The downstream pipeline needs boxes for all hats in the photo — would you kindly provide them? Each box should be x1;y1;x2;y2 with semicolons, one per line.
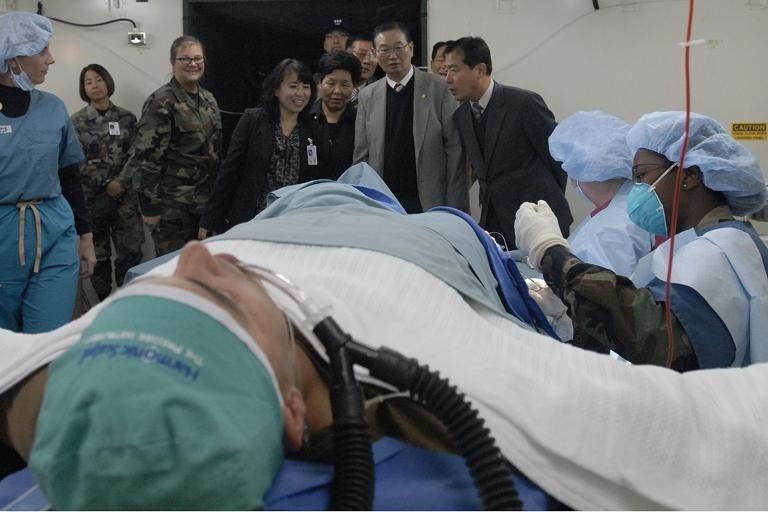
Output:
323;17;353;36
27;283;286;511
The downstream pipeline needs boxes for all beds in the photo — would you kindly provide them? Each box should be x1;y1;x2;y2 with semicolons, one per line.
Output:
0;251;555;511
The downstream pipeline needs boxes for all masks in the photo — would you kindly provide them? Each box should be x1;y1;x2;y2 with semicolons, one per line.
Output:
7;56;34;92
572;180;597;210
626;162;679;239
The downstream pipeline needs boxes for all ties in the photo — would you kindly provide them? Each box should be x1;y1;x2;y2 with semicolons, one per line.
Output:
472;102;483;120
394;83;404;93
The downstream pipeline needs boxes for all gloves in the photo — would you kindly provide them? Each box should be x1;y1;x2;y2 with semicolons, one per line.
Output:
524;277;568;321
514;199;570;274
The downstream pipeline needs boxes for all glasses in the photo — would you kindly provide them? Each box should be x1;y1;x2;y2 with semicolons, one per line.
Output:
352;48;376;59
374;42;410;54
176;55;207;65
325;32;350;40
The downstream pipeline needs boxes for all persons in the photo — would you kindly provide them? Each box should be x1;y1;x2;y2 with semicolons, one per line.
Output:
69;63;146;305
319;16;354;58
430;38;478;222
353;18;462;216
344;30;384;107
548;111;668;282
133;35;224;260
305;50;363;187
0;13;98;335
222;57;327;236
8;238;457;510
442;35;574;252
512;110;768;374
